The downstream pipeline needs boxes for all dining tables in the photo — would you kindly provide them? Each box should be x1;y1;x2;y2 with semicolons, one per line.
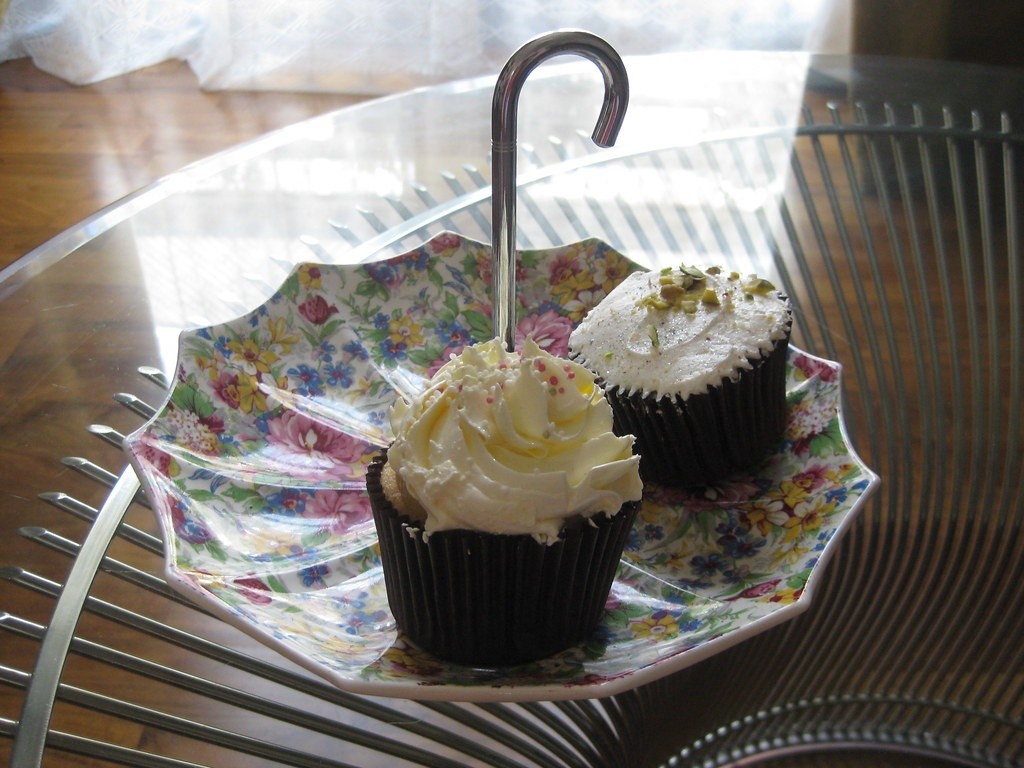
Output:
0;38;1020;766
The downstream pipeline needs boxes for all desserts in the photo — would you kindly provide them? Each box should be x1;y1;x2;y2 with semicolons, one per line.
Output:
566;264;795;490
380;333;643;667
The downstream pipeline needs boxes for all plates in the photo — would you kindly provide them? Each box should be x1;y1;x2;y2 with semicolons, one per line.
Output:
125;221;885;705
